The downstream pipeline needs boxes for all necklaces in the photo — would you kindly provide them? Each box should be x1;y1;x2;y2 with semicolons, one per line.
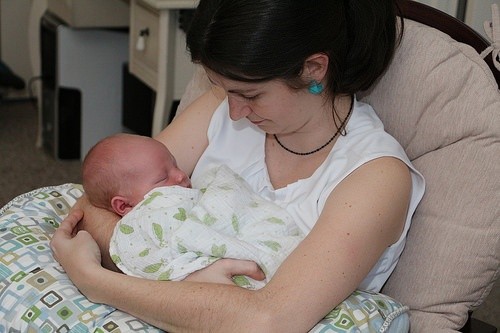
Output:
274;95;355;155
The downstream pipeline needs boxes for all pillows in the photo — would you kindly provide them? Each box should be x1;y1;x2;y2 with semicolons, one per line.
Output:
0;184;411;332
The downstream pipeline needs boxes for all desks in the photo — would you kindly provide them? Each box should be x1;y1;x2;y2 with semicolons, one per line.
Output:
27;0;199;161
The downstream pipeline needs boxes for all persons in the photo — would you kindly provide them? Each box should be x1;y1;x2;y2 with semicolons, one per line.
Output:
49;0;426;333
81;132;308;289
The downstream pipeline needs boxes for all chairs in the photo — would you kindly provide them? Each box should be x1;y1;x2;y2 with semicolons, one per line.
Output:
172;0;500;333
